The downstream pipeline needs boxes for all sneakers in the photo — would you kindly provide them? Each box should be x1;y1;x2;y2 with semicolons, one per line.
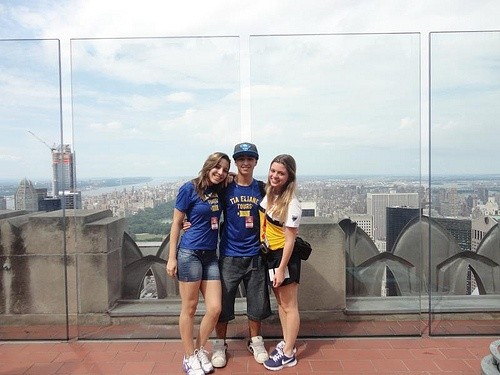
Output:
211;338;228;367
263;349;298;370
182;349;205;375
249;335;268;364
269;340;296;359
195;346;214;373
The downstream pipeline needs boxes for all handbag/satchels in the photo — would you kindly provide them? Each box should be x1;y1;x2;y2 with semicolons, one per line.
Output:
295;237;312;261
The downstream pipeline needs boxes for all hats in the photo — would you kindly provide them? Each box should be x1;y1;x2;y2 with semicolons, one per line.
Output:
233;142;259;159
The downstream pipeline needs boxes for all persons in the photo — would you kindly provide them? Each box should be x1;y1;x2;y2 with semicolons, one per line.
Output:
182;143;298;368
229;154;301;370
166;152;233;375
